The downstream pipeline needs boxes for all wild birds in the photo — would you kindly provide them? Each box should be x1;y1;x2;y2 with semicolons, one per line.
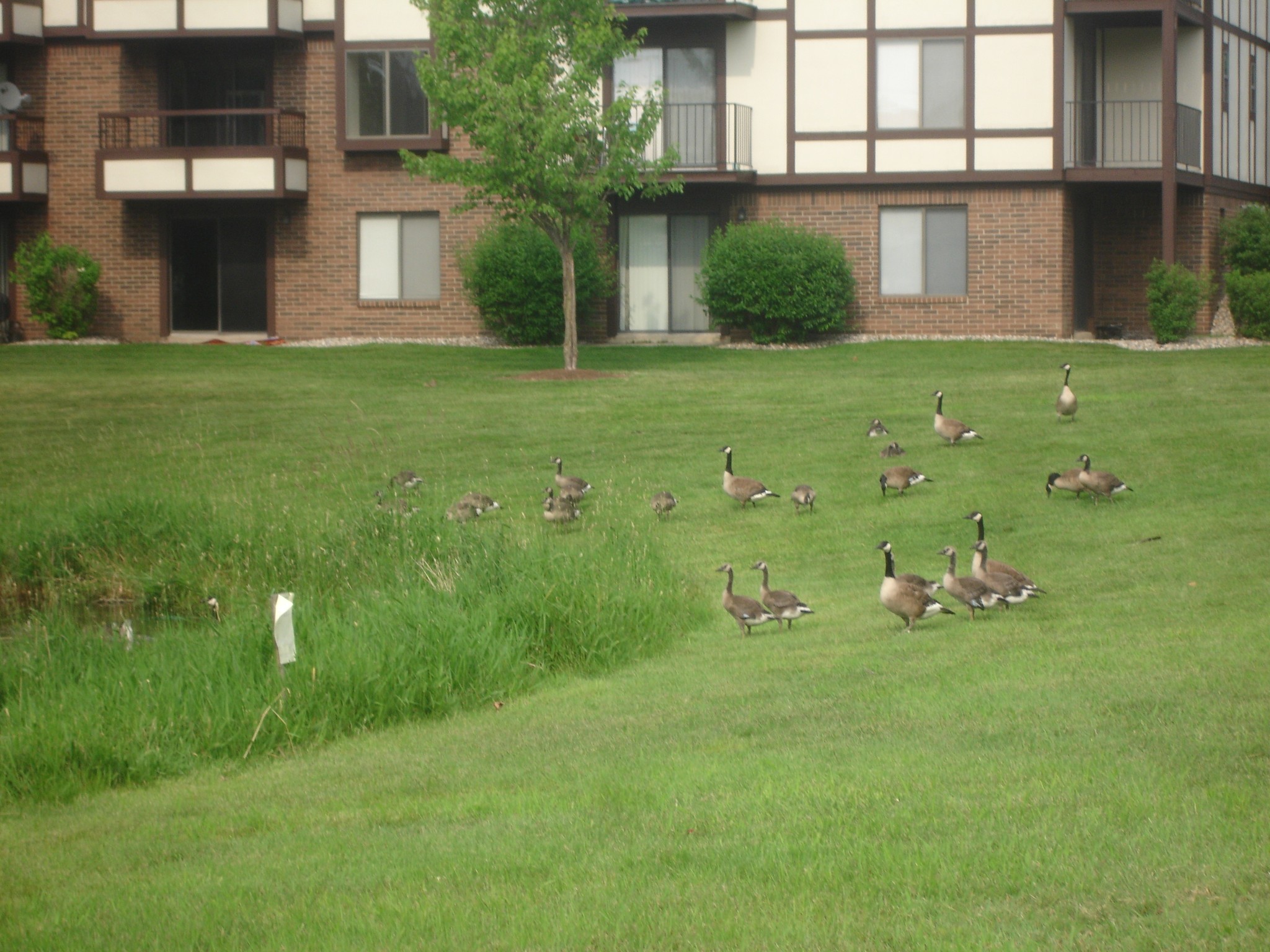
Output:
937;546;1010;623
875;541;955;631
716;564;780;637
1056;363;1078;422
962;511;1047;609
881;442;906;457
374;456;596;526
931;390;984;443
651;490;677;520
866;418;888;436
750;561;815;630
791;485;815;515
1046;454;1134;505
879;466;934;496
719;445;781;509
